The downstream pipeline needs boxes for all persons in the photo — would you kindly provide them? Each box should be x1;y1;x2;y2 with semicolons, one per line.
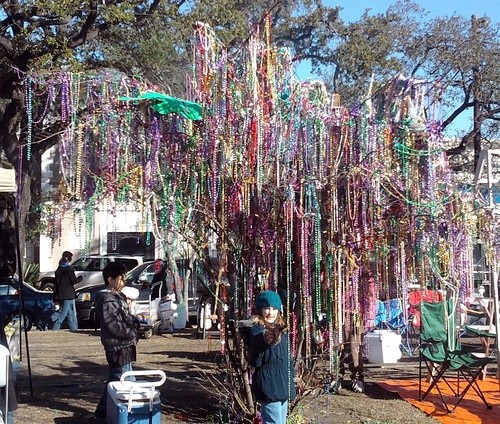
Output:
53;252;85;330
243;292;296;424
95;262;139;419
329;332;372;392
150;259;172;300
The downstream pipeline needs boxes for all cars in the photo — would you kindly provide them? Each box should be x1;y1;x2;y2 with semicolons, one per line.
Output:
0;277;57;331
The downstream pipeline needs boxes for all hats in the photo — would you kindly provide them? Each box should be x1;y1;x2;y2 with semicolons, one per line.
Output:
255;290;282;312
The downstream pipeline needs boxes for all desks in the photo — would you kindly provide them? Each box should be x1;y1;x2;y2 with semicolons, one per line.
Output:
419;344;494;413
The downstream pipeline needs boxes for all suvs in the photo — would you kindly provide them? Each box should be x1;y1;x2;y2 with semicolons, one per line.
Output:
38;254;151;296
76;257;242;330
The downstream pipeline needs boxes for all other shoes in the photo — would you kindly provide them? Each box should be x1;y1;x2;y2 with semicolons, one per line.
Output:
353;380;364;393
325;378;343;394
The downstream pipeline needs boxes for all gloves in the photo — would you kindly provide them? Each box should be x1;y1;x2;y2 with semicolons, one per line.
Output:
264;323;288;345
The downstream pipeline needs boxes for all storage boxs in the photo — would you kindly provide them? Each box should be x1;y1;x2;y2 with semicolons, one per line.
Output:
108;369;166;424
